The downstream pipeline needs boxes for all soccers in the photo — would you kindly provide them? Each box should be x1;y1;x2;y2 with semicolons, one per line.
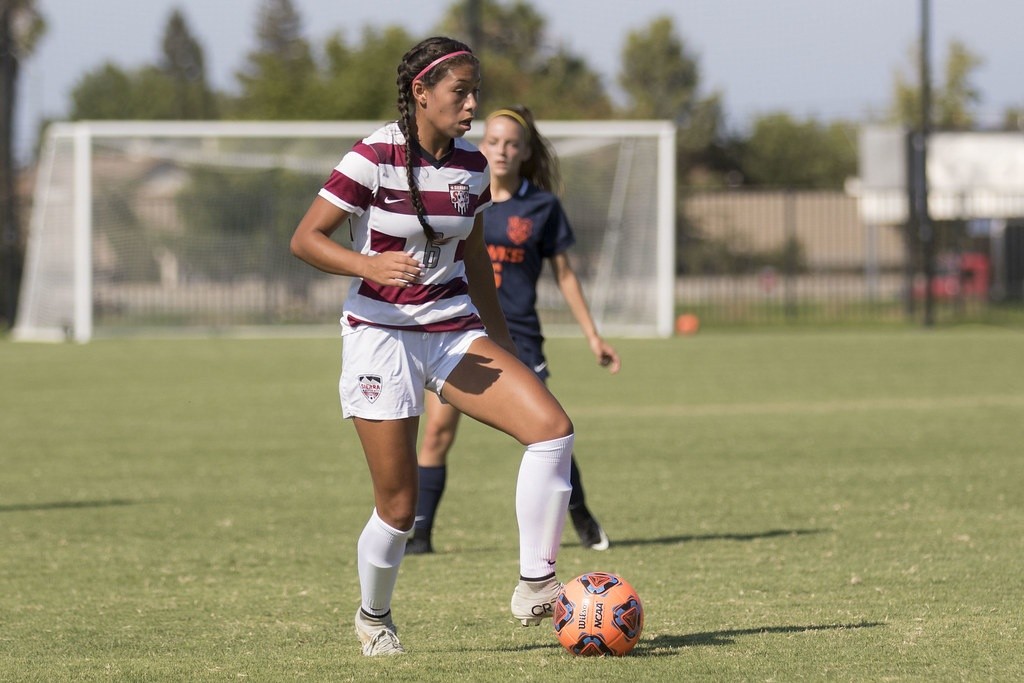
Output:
676;313;699;334
553;571;646;658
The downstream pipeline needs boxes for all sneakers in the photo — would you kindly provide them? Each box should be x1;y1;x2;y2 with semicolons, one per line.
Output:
354;606;404;656
575;516;609;550
405;538;433;555
510;583;567;626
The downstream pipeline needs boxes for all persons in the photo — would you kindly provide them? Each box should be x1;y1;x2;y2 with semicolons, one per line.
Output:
289;36;575;659
403;104;622;558
